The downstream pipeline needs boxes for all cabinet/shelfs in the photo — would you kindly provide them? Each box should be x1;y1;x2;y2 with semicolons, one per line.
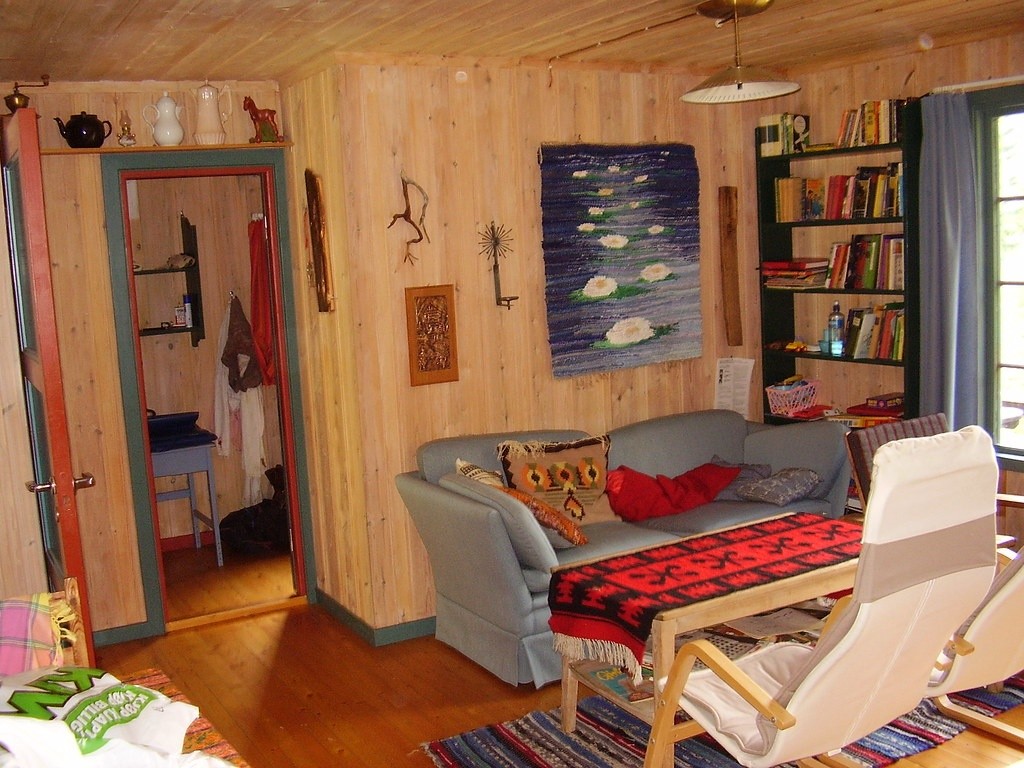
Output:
133;210;206;347
754;91;931;426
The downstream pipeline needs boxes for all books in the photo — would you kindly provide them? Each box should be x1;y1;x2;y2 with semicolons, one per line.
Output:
837;99;905;149
827;390;905;428
826;161;904;221
775;176;825;223
844;302;904;363
826;234;905;292
760;257;828;290
760;112;810;158
805;143;834;151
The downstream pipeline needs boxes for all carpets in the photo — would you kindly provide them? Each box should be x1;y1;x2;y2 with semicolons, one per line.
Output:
418;677;1024;768
114;667;251;768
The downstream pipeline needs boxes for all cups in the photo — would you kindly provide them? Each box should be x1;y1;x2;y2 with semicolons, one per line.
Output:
830;340;843;356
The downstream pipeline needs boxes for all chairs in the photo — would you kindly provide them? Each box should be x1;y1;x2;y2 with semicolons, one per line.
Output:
814;544;1024;768
844;414;1018;548
643;425;1000;768
0;576;89;681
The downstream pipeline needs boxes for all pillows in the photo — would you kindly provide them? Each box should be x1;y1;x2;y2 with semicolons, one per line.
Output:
455;457;590;549
496;433;623;526
655;459;742;514
711;455;772;503
437;471;559;574
737;466;825;509
608;464;677;523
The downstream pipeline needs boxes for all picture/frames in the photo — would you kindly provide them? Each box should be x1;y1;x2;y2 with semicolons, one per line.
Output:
404;284;459;387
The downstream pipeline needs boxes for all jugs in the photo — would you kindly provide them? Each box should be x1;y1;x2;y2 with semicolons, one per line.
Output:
141;91;185;147
188;78;233;145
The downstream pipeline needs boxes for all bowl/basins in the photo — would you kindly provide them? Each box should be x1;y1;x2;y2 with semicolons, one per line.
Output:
818;340;829;354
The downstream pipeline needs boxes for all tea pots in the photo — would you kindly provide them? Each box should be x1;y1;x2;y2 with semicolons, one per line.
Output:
53;111;113;149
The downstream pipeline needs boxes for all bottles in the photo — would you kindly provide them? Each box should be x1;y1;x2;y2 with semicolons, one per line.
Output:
173;294;193;328
829;300;846;356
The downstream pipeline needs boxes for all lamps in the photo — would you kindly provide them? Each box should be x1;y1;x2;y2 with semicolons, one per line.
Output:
678;0;802;106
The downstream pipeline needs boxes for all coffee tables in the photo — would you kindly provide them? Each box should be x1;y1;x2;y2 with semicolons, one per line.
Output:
546;510;863;768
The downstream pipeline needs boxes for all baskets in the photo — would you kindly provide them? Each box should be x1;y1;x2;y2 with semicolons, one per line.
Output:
765;379;821;416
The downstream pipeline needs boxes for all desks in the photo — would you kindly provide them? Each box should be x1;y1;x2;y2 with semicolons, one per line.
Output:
151;443;224;568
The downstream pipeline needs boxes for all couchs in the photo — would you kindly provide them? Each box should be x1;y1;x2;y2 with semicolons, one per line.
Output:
394;409;852;691
